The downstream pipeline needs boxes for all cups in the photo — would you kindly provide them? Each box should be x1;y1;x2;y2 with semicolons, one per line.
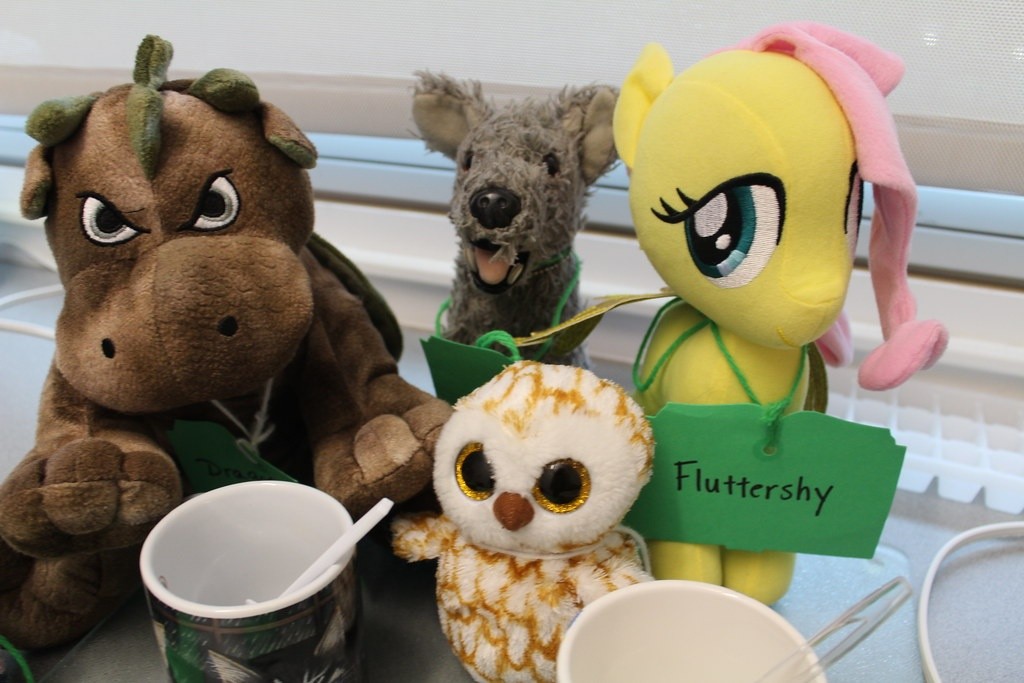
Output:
557;580;826;683
140;479;355;683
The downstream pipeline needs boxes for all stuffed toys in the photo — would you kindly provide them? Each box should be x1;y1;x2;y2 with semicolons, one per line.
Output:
393;360;653;683
611;24;947;611
0;34;456;651
414;71;622;368
